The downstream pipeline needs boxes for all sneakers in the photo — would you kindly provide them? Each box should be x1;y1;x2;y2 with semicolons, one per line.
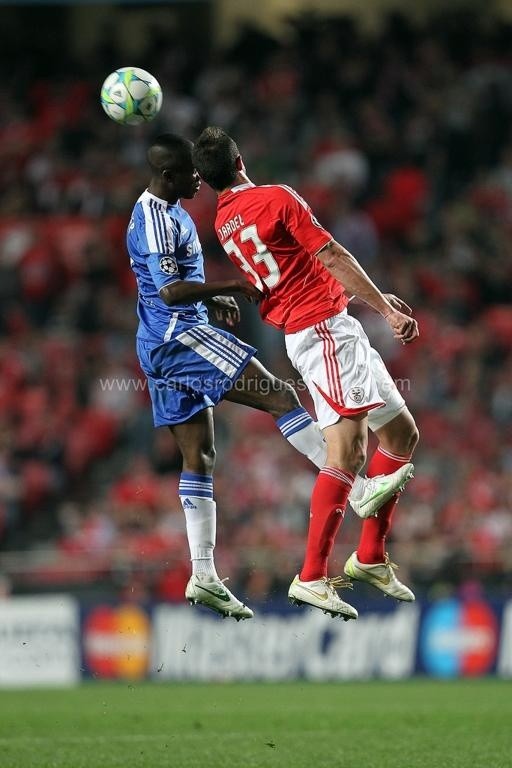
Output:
344;551;415;602
185;575;253;621
351;463;415;518
287;574;358;622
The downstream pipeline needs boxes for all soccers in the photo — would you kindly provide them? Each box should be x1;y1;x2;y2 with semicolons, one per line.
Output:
101;67;162;127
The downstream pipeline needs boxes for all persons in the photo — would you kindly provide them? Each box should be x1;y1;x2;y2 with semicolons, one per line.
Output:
189;125;420;618
126;134;414;619
